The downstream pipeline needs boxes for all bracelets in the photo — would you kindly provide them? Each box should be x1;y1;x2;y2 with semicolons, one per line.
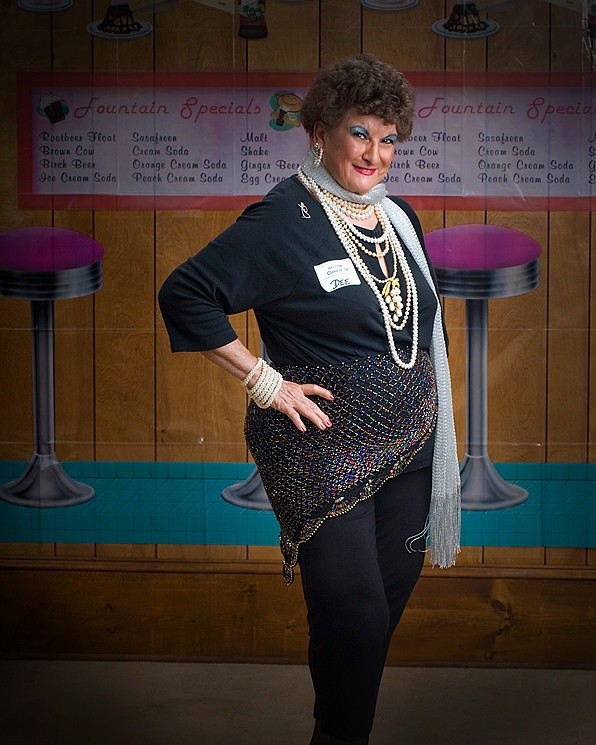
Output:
241;358;284;408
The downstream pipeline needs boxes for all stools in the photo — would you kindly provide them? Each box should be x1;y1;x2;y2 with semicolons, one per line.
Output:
426;220;540;514
0;226;103;508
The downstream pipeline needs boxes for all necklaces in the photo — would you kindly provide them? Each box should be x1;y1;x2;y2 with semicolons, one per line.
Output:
298;162;419;370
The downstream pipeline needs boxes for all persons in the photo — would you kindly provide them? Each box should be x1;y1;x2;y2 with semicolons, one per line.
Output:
159;54;463;745
